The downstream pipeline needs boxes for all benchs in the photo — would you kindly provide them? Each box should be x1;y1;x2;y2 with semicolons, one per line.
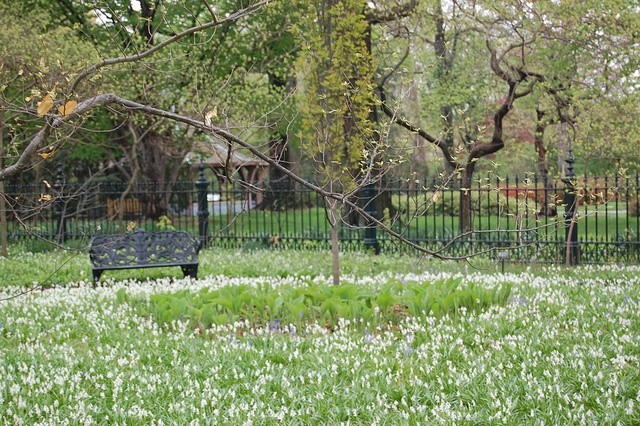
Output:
88;232;202;287
